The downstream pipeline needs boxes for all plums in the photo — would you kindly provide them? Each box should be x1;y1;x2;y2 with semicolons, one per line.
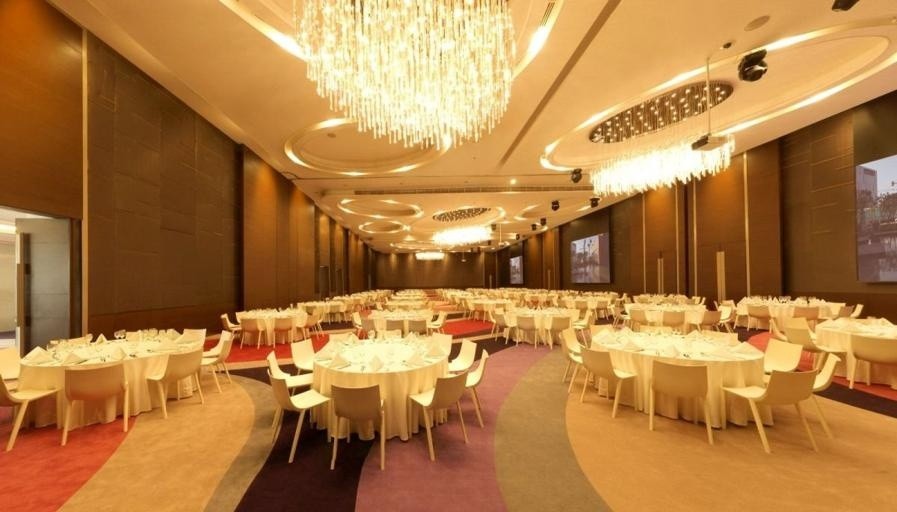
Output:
691;135;728;151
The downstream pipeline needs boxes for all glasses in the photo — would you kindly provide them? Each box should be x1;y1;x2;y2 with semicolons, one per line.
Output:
291;0;517;151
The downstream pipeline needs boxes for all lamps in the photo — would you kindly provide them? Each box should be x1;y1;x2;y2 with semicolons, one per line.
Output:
290;339;315;376
361;318;374;335
556;332;584;395
649;359;713;445
516;316;540;349
629;310;652;329
691;311;721;332
747;303;776;333
563;327;586;354
0;374;61;452
220;313;242;342
273;317;293;349
267;350;313;429
544;316;571;350
145;349;204;420
239;318;266;350
849;334;897;390
580;345;638;418
199;341;232;393
785;328;847;370
428;311;447;335
662;310;685;330
446;349;490;428
329;384;385;470
493;313;517;345
295;316;319;341
408;319;425;336
720;368;819;453
266;369;332;463
446;336;477;373
408;369;469;461
386;318;404;336
60;362;128;446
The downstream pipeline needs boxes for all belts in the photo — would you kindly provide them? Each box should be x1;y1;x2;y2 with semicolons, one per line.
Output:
366;329;376;342
119;329;126;340
45;338;67;360
137;327;175;347
113;330;121;342
